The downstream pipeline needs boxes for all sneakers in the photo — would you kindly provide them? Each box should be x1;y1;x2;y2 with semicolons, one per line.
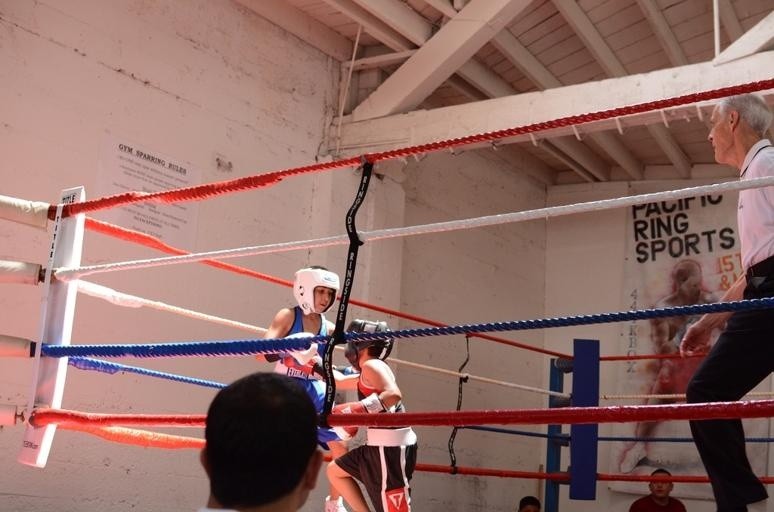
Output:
325;494;347;512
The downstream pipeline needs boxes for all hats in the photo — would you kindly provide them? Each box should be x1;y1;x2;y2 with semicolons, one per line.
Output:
204;372;330;453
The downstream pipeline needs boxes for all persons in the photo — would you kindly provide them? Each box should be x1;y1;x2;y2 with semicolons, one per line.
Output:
192;372;324;512
620;260;725;477
685;94;774;512
519;496;540;512
628;468;687;512
256;265;358;512
326;319;418;512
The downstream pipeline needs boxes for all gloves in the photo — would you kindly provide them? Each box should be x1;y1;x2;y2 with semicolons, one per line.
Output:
331;392;385;440
285;332;318;365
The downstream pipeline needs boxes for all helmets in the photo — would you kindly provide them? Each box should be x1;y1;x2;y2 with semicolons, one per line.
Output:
293;266;340;316
345;319;393;370
